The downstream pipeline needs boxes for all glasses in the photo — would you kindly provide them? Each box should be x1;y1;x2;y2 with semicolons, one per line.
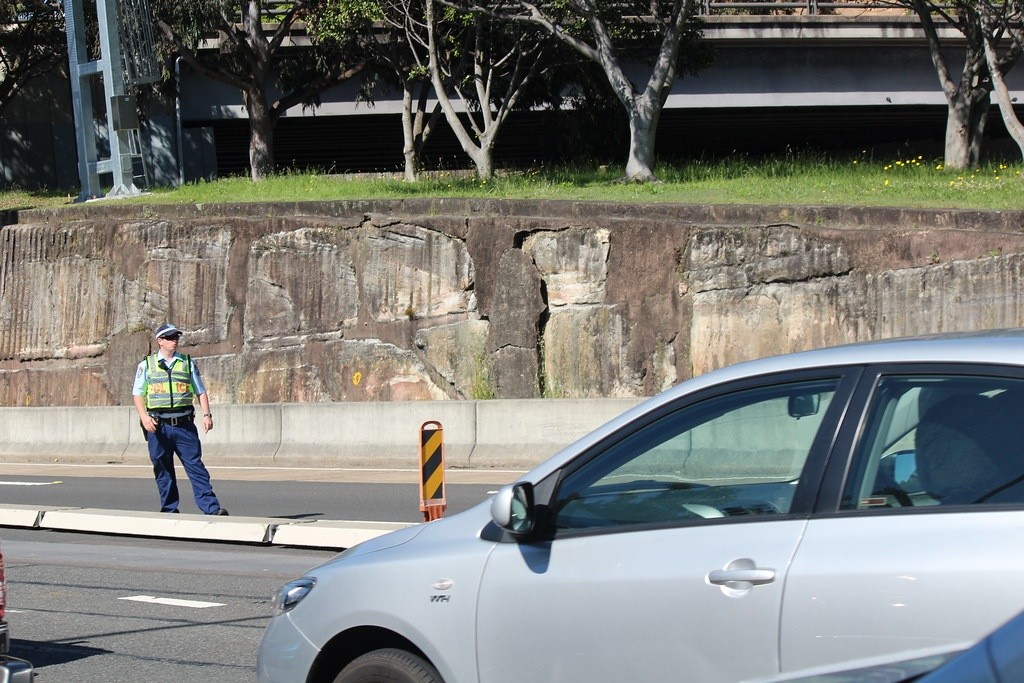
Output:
164;335;180;340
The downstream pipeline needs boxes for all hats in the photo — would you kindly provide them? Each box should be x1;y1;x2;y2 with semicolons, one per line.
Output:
155;324;182;339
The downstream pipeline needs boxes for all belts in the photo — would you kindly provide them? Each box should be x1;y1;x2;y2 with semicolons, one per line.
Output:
158;415;191;426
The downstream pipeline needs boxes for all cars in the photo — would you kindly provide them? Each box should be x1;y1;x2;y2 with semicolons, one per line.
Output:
254;328;1022;682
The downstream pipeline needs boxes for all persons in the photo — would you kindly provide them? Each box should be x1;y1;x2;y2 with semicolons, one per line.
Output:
132;324;229;516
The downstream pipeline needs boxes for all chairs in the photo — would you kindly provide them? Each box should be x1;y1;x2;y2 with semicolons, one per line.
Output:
915;395;1024;505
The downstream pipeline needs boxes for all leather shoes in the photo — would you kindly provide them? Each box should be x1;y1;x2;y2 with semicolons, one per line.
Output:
214;508;228;516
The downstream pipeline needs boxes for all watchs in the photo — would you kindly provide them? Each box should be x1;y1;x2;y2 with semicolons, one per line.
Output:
204;413;212;418
